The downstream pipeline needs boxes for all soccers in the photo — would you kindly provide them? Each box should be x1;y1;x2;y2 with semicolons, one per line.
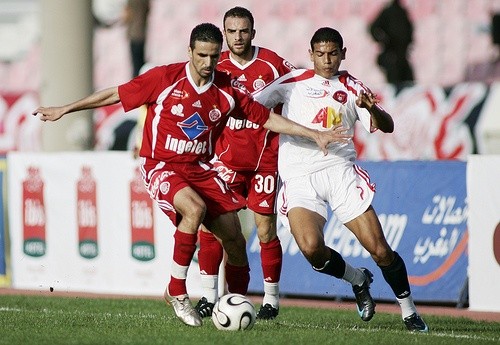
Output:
212;293;256;331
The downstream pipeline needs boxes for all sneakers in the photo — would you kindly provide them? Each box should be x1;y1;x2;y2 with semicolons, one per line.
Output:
163;286;202;327
351;267;377;323
256;304;279;320
401;313;428;334
195;296;215;318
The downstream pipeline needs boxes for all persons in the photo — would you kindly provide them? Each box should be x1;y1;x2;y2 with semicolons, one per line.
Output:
464;13;500;84
371;0;415;97
192;7;297;319
31;23;354;328
249;27;429;332
120;0;149;78
